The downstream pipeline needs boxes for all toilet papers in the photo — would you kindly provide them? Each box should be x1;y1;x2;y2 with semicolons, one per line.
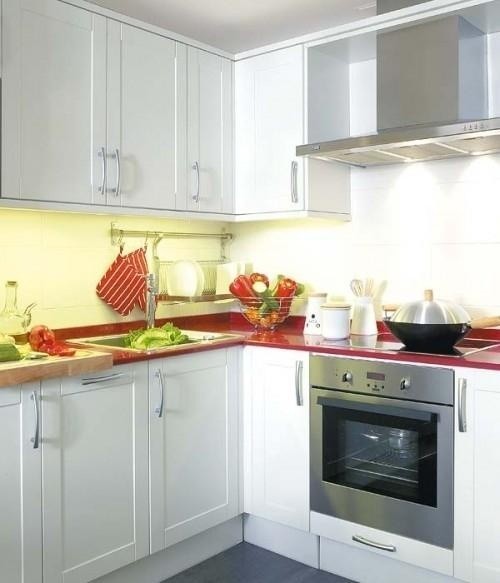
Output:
214;261;239;297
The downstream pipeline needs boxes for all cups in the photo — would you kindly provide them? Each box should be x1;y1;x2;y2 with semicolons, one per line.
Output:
302;292;327;337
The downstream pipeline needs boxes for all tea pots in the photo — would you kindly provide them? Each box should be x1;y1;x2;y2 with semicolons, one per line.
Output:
0;280;37;334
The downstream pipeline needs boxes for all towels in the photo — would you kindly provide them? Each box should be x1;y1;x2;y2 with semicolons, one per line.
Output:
96;252;146;317
125;248;159;310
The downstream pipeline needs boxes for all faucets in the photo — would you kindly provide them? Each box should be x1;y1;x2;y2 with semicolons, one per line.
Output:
144;272;159;331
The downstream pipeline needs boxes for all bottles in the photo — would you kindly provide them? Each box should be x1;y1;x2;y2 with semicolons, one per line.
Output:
321;302;351;340
348;296;378;335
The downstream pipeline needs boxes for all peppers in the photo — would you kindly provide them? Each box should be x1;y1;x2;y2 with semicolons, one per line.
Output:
230;273;296;317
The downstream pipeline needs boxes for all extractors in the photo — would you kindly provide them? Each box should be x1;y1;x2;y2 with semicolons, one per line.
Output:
297;14;500;170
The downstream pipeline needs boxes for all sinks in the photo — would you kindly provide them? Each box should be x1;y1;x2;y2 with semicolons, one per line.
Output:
74;326;229;353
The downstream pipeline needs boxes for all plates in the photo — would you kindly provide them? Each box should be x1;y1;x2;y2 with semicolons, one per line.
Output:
166;257;206;304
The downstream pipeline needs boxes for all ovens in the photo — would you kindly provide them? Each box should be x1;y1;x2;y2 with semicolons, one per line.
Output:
309;386;455;583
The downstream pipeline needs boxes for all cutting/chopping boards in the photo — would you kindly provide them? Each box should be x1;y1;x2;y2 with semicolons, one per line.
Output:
0;343;113;389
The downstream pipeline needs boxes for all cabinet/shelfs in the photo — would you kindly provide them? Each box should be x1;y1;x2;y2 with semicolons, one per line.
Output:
0;0;188;213
229;39;351;225
177;32;234;221
310;509;454;581
242;343;311;534
453;363;500;582
41;361;150;583
147;344;245;557
0;378;44;583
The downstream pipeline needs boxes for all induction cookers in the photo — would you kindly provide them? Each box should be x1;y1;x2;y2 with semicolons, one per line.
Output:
308;330;499;405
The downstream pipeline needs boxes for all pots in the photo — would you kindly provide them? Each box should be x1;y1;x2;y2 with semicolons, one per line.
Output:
382;288;499;351
